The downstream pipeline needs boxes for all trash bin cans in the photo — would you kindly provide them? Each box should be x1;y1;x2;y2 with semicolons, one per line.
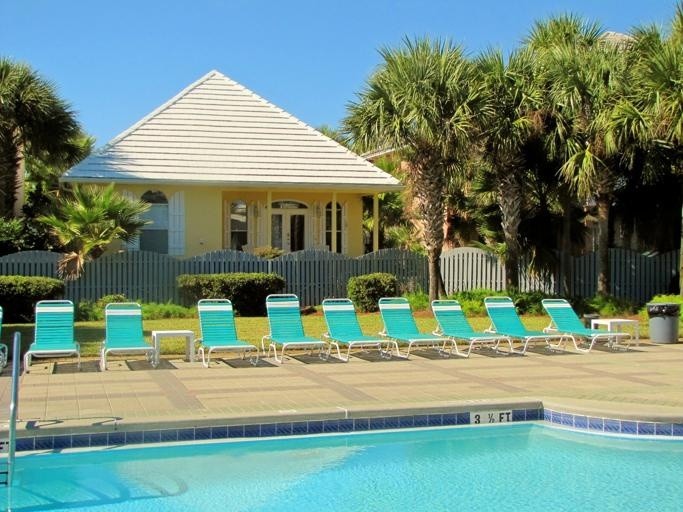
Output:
646;303;681;344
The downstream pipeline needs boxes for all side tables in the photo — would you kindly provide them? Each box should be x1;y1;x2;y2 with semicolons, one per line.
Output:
152;330;193;364
591;318;639;347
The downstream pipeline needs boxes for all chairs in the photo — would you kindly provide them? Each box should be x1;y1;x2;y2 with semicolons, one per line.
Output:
482;296;568;355
431;300;512;357
100;303;154;370
261;293;321;364
0;306;9;375
319;298;389;362
24;299;81;373
197;299;259;368
378;296;454;359
541;298;632;352
